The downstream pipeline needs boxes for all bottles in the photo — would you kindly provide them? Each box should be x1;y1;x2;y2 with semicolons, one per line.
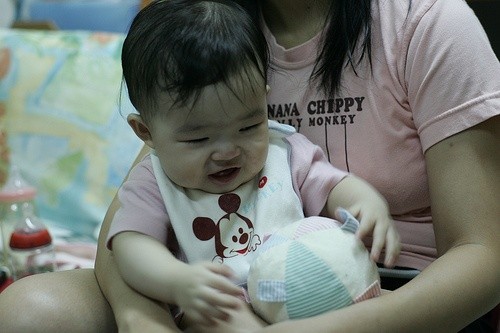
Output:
0;165;39;272
9;204;58;282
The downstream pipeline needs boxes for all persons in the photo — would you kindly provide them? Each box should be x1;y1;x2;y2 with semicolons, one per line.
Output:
106;2;403;327
0;0;500;333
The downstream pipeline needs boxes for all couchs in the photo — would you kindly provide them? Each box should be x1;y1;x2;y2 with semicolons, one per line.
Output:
1;29;145;278
